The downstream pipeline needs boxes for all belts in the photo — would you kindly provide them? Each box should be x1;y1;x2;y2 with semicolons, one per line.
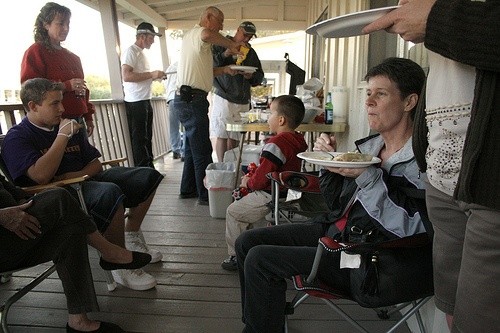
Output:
191;90;207;96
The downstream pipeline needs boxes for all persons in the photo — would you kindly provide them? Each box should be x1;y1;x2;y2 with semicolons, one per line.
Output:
175;6;248;205
361;0;500;333
212;20;264;173
21;2;96;141
235;58;434;333
165;60;187;162
1;77;162;291
121;23;166;178
0;176;151;333
221;95;308;270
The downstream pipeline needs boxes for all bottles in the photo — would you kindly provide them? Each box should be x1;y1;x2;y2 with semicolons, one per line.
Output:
236;46;250;65
324;92;333;124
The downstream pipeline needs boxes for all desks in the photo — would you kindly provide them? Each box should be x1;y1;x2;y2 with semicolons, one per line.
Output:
226;123;349;202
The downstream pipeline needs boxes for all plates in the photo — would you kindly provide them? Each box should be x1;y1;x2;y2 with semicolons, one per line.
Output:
304;5;401;38
297;150;382;168
227;65;258;74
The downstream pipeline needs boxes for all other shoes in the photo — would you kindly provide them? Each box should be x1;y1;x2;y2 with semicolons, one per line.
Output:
66;318;125;333
181;156;185;161
173;152;181;159
199;198;209;206
99;249;153;271
180;193;199;198
220;255;238;271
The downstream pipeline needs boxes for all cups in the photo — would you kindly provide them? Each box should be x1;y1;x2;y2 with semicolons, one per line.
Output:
75;79;86;99
248;108;261;123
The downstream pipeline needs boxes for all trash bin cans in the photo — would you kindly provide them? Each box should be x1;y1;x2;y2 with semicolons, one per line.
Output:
207;161;237;219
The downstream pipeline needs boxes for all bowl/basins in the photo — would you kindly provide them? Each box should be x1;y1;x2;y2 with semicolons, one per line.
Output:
240;111;248;117
301;108;317;124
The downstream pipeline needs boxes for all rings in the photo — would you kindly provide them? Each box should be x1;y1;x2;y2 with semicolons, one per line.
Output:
77;84;78;88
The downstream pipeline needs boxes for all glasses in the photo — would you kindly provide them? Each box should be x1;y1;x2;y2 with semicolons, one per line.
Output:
241;32;254;39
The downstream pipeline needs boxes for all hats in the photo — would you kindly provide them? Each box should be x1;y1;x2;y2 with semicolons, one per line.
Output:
136;22;162;37
240;21;258;40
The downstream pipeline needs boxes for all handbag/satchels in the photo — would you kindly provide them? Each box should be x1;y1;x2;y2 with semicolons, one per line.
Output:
313;237;376;303
359;232;437;309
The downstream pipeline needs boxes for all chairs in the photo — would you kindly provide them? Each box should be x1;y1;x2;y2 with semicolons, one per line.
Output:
0;135;129;333
267;169;434;333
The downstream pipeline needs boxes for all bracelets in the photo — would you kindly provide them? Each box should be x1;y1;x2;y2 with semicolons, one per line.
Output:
57;133;72;140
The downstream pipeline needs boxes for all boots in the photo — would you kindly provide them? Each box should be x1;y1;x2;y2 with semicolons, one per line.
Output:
125;230;162;264
111;255;157;292
